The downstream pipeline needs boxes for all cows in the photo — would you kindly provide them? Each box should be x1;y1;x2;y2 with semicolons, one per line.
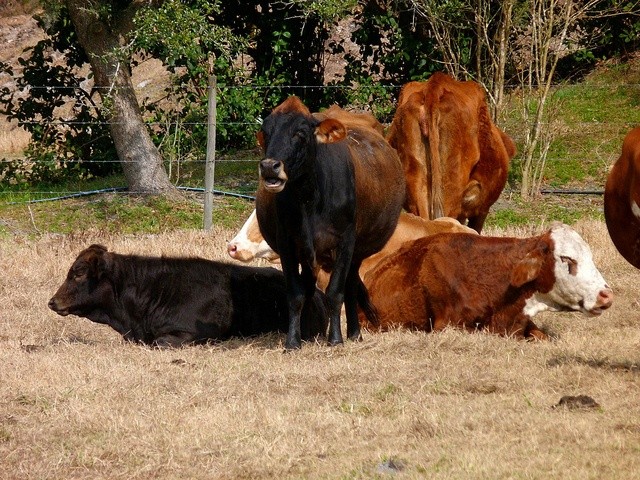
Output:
385;70;519;235
47;243;330;351
356;220;614;346
254;94;408;358
226;203;483;295
